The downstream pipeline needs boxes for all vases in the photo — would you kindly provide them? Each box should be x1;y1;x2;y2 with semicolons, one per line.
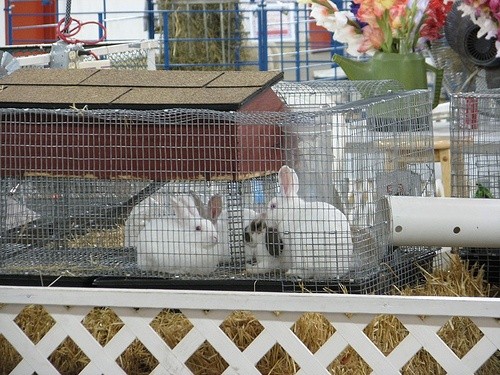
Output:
332;51;445;115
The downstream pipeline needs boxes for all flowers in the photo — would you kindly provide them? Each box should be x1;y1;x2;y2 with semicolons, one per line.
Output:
305;0;455;55
458;2;499;61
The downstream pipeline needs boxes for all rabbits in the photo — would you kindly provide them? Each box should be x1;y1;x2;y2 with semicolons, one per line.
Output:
190;189;265;264
123;191;222;276
245;212;285;273
263;165;354;279
